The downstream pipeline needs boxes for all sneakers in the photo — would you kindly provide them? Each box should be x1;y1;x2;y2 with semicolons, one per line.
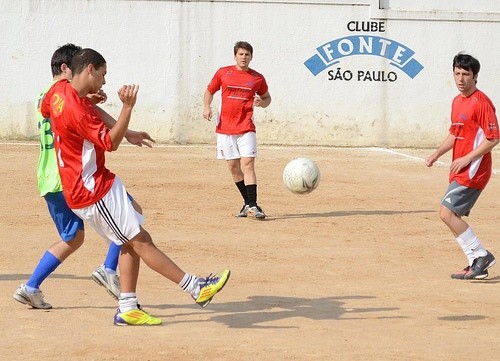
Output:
451;266;488;279
235;205;265;219
91;263;120;300
114;305;163;326
13;284;52;309
464;250;496;279
196;268;231;308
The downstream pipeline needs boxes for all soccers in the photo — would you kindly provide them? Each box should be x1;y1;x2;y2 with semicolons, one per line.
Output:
282;157;321;196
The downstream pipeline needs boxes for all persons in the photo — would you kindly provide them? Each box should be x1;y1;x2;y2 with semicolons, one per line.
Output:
203;42;271;218
13;43;230;326
425;53;500;278
